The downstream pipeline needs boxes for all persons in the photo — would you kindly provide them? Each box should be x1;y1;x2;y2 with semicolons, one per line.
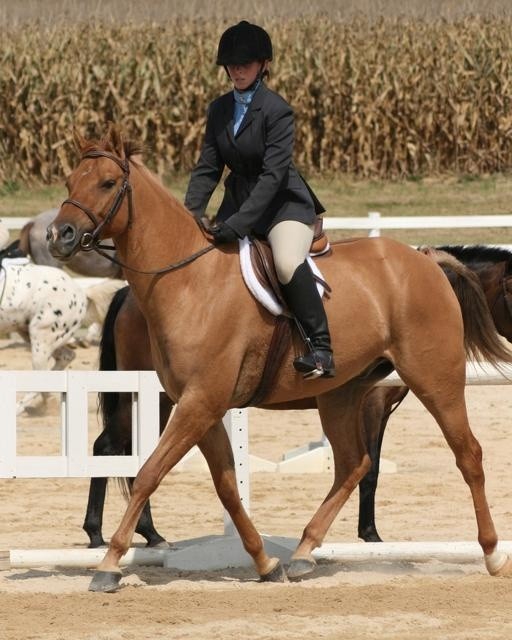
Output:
184;19;336;378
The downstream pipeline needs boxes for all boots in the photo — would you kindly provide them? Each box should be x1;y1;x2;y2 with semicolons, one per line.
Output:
278;259;336;379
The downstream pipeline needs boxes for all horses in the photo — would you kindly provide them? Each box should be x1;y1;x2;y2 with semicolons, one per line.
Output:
46;123;512;593
0;205;129;416
84;244;512;550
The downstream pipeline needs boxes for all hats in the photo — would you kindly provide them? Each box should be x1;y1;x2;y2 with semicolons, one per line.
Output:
215;20;273;67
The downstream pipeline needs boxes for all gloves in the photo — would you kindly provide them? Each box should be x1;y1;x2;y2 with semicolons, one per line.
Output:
207;224;240;242
